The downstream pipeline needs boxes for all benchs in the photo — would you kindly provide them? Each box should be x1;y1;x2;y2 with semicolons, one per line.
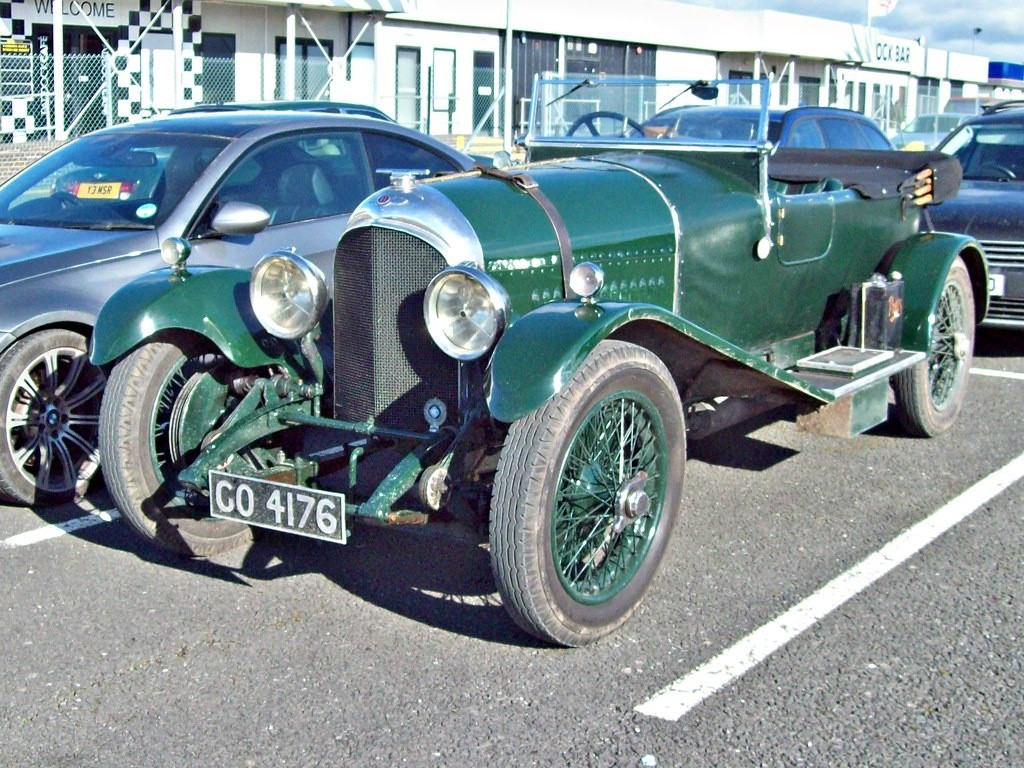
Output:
764;146;962;210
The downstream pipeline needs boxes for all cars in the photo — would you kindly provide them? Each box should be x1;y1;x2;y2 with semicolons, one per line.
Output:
1;101;522;507
89;75;990;648
621;98;1024;330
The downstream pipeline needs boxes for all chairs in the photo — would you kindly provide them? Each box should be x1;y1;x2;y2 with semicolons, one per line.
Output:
267;164;335;229
151;145;206;228
